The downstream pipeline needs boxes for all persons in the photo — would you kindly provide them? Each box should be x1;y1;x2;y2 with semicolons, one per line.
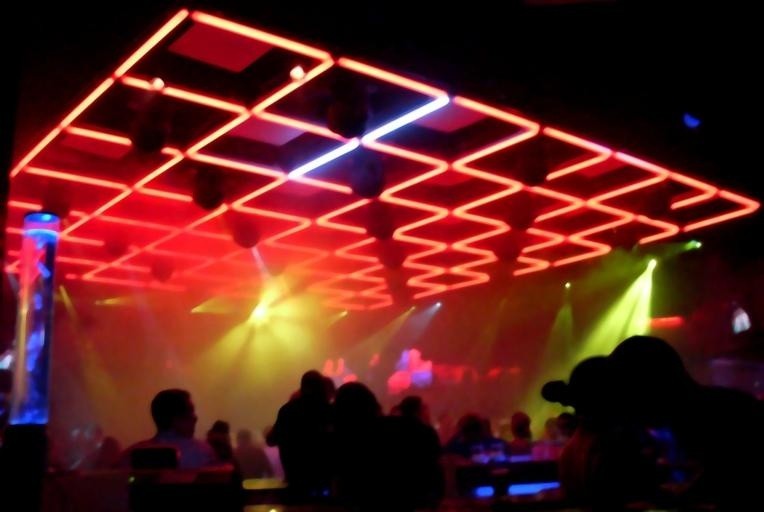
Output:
231;423;273;494
101;388;220;512
435;409;575;459
541;335;764;512
267;370;447;512
204;420;233;463
